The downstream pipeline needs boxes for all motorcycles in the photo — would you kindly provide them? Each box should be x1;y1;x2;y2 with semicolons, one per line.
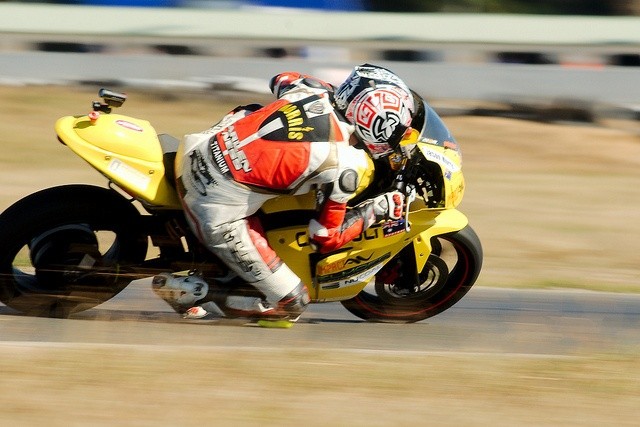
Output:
0;89;482;324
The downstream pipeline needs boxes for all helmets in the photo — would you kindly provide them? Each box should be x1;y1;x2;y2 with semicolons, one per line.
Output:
333;65;415;159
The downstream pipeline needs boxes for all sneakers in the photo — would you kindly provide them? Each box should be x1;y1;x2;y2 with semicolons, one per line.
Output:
152;270;209;318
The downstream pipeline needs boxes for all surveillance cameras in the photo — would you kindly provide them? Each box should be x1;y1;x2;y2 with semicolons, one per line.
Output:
99;88;128;107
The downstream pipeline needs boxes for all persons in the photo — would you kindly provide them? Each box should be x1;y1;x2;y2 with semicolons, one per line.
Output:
152;62;416;322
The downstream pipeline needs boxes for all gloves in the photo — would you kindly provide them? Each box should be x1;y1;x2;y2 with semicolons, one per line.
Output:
353;187;405;231
